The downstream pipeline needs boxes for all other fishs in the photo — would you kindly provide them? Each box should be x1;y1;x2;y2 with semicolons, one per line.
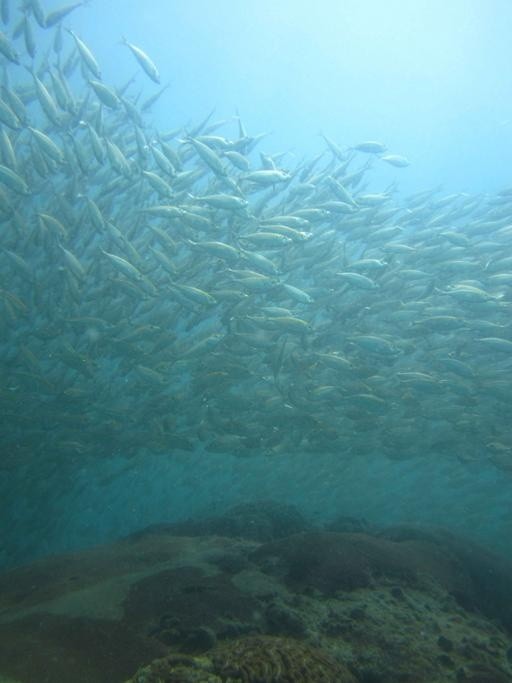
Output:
0;0;511;570
